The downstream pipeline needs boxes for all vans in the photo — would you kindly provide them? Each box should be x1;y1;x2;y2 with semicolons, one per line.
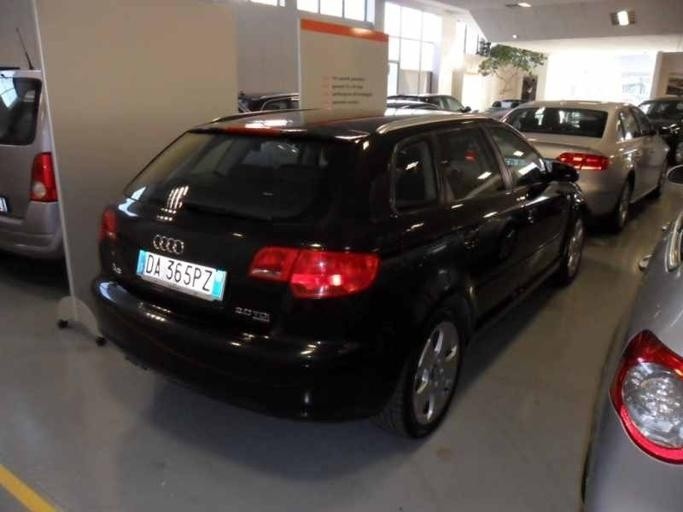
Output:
1;69;64;259
88;109;593;438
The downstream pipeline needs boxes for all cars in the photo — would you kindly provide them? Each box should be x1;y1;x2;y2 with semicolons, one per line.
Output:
386;94;522;120
579;165;683;512
500;95;683;233
